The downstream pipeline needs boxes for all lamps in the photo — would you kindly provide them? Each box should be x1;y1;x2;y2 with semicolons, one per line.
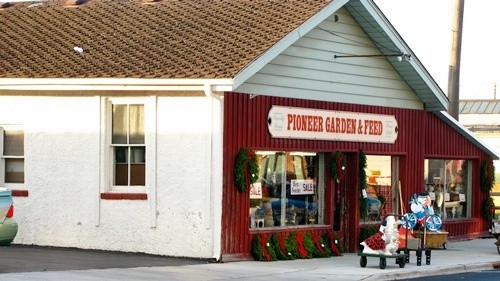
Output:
397;53;412;62
